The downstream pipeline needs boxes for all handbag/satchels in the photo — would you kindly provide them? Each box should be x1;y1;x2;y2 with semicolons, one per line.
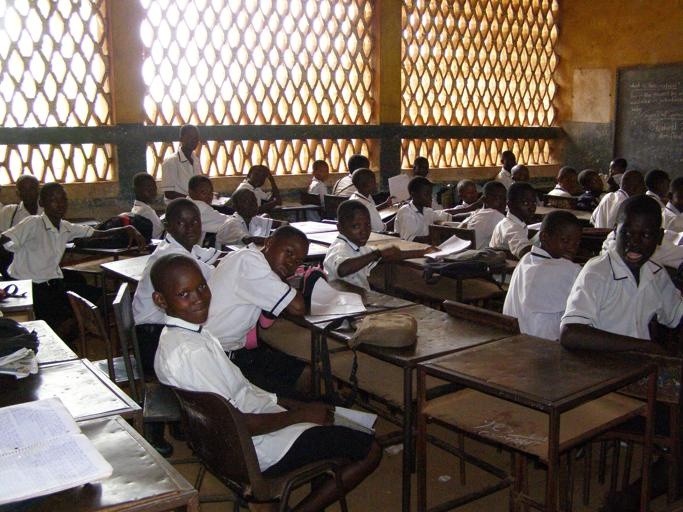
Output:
346;312;419;351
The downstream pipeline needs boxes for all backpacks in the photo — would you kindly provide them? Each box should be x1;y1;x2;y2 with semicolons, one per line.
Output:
72;213;154;250
421;246;510;293
244;264;329;351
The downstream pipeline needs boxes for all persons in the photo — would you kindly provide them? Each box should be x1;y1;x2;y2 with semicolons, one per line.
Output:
0;124;683;512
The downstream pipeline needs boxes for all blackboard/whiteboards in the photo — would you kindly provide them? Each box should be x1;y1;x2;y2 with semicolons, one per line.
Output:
613;63;683;198
